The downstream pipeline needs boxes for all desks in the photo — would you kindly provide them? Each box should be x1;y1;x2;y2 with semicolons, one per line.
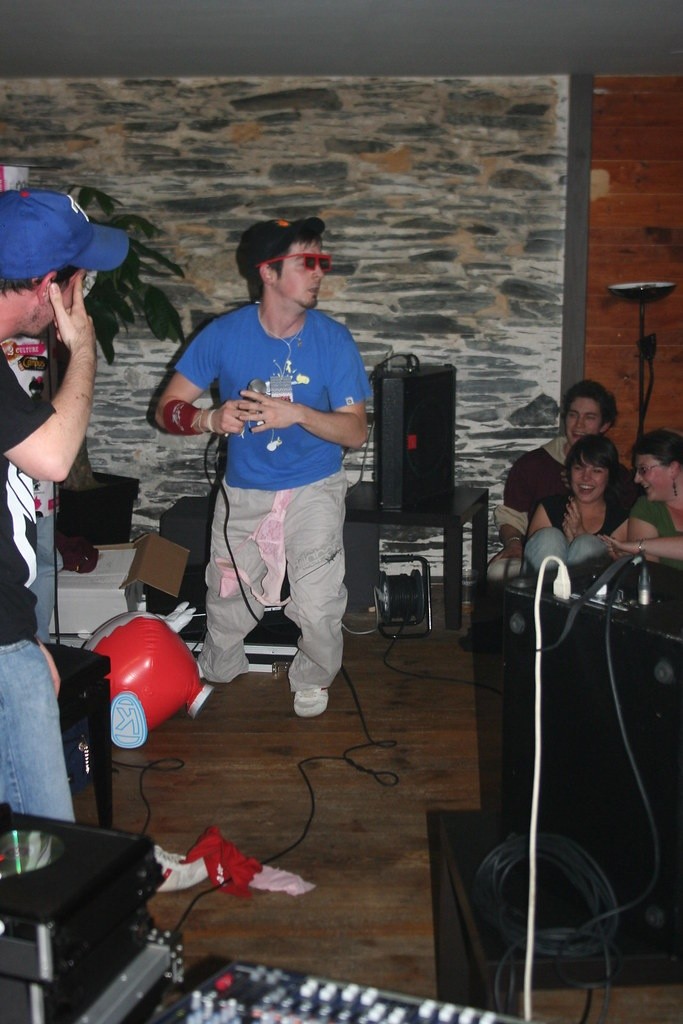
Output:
42;642;114;830
343;481;488;632
425;807;683;1017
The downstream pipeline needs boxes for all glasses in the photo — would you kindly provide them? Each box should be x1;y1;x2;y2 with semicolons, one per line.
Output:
635;462;670;476
256;253;332;273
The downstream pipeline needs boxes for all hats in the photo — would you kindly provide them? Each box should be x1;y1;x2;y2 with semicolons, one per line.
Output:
0;188;130;279
236;217;325;279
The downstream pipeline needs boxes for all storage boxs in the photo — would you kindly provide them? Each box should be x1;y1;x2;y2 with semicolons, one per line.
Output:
49;532;190;634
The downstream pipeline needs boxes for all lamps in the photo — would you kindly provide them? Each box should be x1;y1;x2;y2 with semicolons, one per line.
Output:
608;281;677;436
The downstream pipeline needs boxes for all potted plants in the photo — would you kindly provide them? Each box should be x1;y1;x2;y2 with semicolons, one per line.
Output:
55;183;187;545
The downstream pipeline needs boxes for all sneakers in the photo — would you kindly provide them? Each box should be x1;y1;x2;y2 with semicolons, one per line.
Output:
139;845;210;892
293;686;329;716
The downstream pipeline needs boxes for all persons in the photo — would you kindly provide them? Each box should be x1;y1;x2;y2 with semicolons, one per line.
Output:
0;187;129;823
487;380;683;596
156;216;374;717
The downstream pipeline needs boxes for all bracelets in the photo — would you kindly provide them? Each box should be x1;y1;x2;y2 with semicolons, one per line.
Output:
639;537;646;552
191;408;216;433
505;537;521;546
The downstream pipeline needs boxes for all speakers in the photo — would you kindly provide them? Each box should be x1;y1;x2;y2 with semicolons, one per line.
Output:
426;806;683;1017
373;365;458;513
498;584;683;824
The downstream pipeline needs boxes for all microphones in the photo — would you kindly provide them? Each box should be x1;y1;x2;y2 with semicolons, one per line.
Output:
213;379;268;434
